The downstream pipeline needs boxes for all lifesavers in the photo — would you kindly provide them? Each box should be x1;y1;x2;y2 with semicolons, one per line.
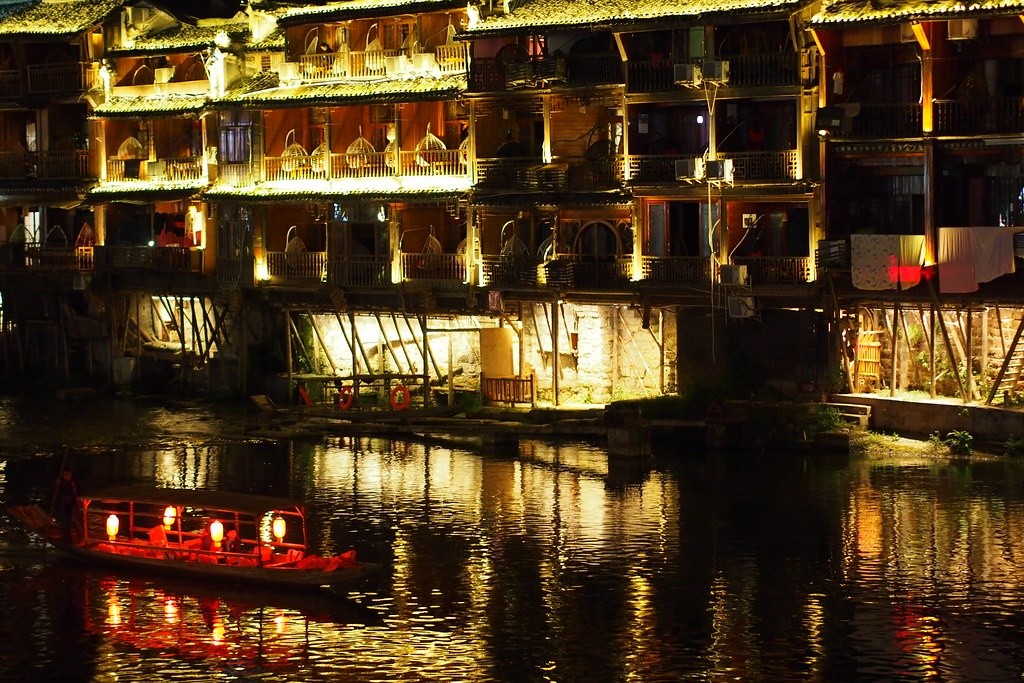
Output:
299;388;315;406
390;386;411;410
339;387;352;408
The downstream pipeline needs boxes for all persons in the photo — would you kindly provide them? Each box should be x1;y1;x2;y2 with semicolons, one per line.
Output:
56;467;84;544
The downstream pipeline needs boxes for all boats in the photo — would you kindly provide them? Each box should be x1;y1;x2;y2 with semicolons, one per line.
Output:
4;488;370;587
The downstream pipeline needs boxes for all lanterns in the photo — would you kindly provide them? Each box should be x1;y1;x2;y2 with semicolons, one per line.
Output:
211;520;224;547
274;516;286;543
107;515;120;542
163;506;177;529
222;529;242;553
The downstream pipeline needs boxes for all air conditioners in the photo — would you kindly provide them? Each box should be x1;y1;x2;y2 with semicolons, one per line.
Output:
705;158;733;180
153;68;174;83
413;53;434;72
279;62;300;80
673;64;703;85
702;60;729;82
72;275;91;289
674;158;703;178
385;55;407;74
727;296;757;318
720;264;748;285
